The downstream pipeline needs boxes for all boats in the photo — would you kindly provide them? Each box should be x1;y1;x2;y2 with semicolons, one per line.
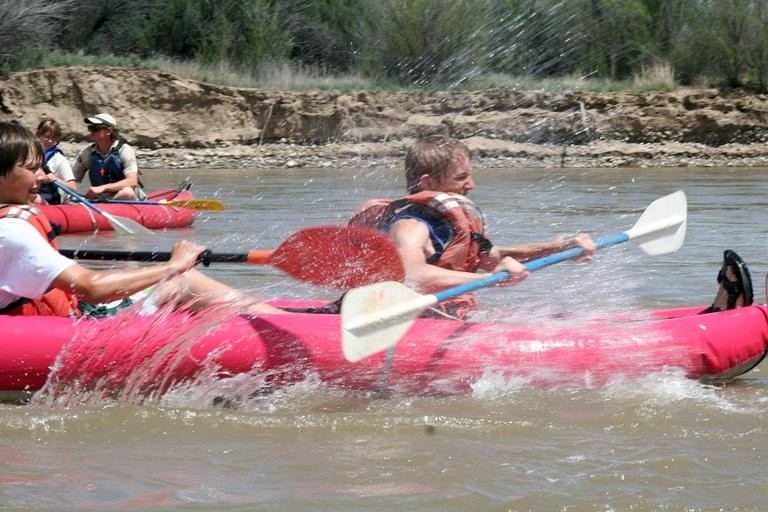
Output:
0;299;768;393
34;177;197;235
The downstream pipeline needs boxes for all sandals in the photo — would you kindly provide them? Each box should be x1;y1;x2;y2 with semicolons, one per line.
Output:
707;249;755;316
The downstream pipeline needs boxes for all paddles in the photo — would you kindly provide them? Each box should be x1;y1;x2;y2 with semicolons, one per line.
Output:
51;177;151;235
340;191;687;364
59;224;405;290
72;199;226;211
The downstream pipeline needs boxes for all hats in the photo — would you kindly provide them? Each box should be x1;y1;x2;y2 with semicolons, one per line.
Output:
83;112;117;129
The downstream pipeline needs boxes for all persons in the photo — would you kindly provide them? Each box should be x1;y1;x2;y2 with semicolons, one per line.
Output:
0;120;292;324
31;118;78;207
70;114;149;201
343;134;755;323
